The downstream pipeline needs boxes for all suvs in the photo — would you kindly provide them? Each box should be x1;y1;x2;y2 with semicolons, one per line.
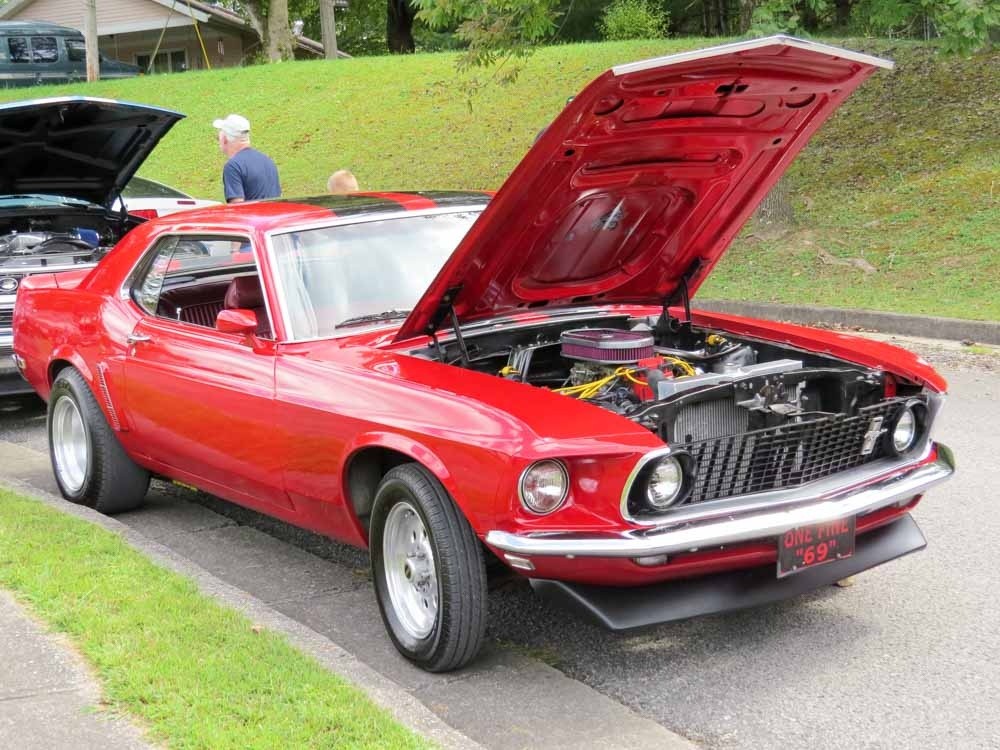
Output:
0;19;146;89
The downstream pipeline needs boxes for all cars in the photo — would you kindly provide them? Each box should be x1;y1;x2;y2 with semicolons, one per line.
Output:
0;95;214;417
110;174;238;258
19;33;957;673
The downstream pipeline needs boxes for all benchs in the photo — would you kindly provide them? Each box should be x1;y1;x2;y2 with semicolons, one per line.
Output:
156;281;226;328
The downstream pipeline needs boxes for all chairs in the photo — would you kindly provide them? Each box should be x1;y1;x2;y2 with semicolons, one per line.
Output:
225;275;270;339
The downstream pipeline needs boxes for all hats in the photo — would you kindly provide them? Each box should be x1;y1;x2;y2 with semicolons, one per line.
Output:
213;114;250;136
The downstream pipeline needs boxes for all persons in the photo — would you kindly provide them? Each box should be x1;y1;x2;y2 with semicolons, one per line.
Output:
328;169;359;196
212;114;283;201
